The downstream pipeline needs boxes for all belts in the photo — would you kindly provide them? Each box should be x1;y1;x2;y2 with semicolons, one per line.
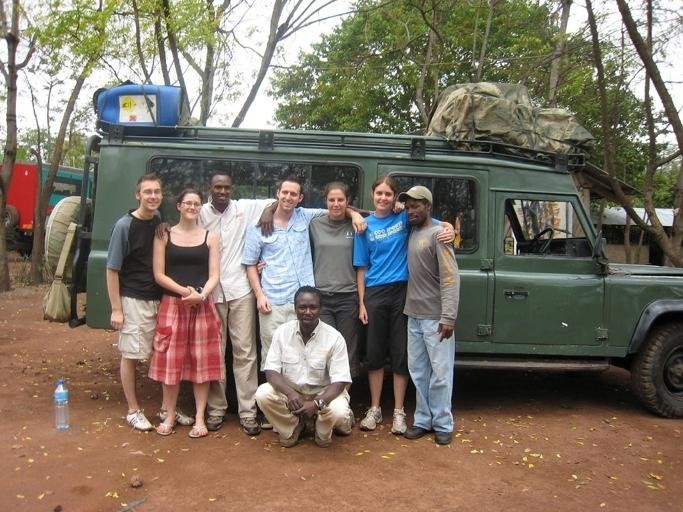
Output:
320;291;336;296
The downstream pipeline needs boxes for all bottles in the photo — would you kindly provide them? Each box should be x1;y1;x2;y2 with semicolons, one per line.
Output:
54;379;70;430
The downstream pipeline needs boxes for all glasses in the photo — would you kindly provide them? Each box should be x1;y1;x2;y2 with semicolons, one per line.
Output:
180;201;202;208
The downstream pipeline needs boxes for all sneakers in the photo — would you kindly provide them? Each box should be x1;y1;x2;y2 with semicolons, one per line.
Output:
278;417;306;447
239;418;261;435
207;416;225;431
405;426;431;439
261;414;273;429
435;429;453;445
359;406;383;430
337;409;354;434
158;406;195;426
391;407;407;434
314;418;332;447
127;409;152;431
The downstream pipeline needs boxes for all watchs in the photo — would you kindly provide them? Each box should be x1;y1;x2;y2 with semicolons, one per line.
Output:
201;294;206;304
314;397;327;410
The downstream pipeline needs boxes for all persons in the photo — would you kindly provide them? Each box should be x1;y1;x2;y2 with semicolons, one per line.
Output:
152;188;222;438
254;286;354;449
106;172;195;432
241;178;368;430
156;173;279;436
353;176;455;434
255;182;405;435
347;186;460;445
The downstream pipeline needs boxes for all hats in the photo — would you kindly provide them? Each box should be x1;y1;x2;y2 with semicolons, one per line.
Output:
398;184;433;205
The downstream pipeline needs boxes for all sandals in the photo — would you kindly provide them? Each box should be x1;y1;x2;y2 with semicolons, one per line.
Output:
189;424;206;438
157;421;177;435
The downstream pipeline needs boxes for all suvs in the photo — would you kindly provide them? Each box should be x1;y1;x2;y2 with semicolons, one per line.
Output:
44;119;683;420
0;162;94;250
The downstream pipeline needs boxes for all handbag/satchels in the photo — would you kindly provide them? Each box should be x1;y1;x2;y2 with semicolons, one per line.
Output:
42;222;77;324
